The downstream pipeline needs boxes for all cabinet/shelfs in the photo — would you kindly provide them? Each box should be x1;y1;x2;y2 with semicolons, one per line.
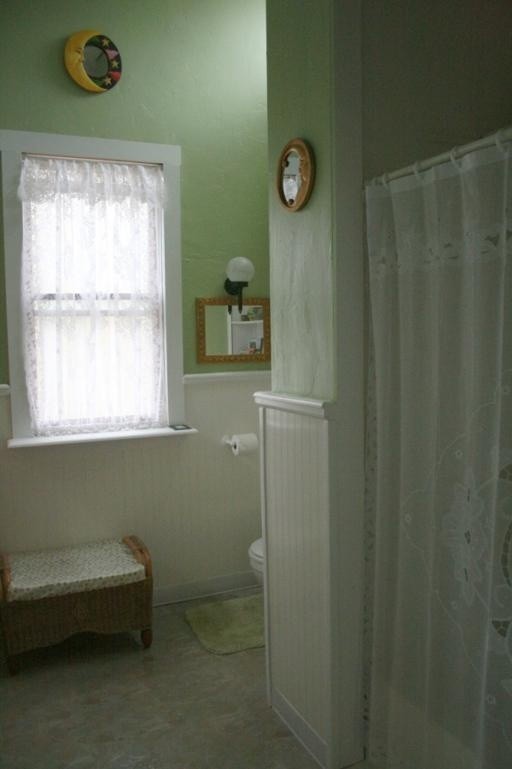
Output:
227;305;264;355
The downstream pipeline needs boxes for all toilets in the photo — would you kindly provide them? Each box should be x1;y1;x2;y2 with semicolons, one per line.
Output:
248;538;264;585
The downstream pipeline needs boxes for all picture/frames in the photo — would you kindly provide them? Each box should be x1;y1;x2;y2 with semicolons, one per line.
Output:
275;138;314;214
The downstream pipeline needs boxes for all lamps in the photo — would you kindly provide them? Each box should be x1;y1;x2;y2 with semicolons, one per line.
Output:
224;257;255;313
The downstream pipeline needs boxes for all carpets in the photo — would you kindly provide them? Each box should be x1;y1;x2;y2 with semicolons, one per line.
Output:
185;591;265;656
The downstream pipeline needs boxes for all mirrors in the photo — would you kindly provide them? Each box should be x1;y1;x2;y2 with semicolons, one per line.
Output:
195;296;271;363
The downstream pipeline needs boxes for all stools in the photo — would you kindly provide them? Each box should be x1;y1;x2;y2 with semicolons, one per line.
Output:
0;535;154;678
